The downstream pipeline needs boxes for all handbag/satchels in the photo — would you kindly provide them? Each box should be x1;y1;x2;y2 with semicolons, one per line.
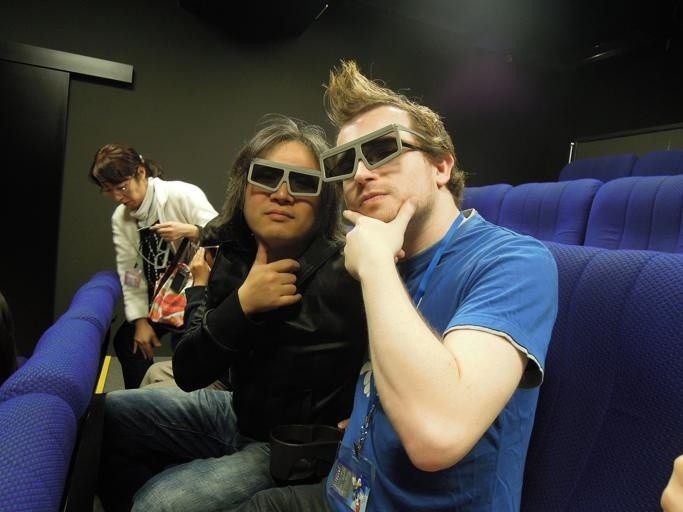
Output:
147;262;193;328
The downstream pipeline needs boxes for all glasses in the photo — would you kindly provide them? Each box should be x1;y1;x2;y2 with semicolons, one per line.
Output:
97;165;139;199
131;225;171;269
318;120;440;182
244;157;324;197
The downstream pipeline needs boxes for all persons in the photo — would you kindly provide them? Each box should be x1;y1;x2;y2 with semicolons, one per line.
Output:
659;455;683;512
138;214;230;389
232;56;560;512
89;142;220;389
88;112;368;512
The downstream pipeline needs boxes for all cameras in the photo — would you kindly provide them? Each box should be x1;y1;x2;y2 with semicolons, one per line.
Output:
170;267;190;294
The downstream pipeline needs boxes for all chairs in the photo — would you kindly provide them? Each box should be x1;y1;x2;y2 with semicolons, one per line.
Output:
462;148;682;512
0;271;120;512
